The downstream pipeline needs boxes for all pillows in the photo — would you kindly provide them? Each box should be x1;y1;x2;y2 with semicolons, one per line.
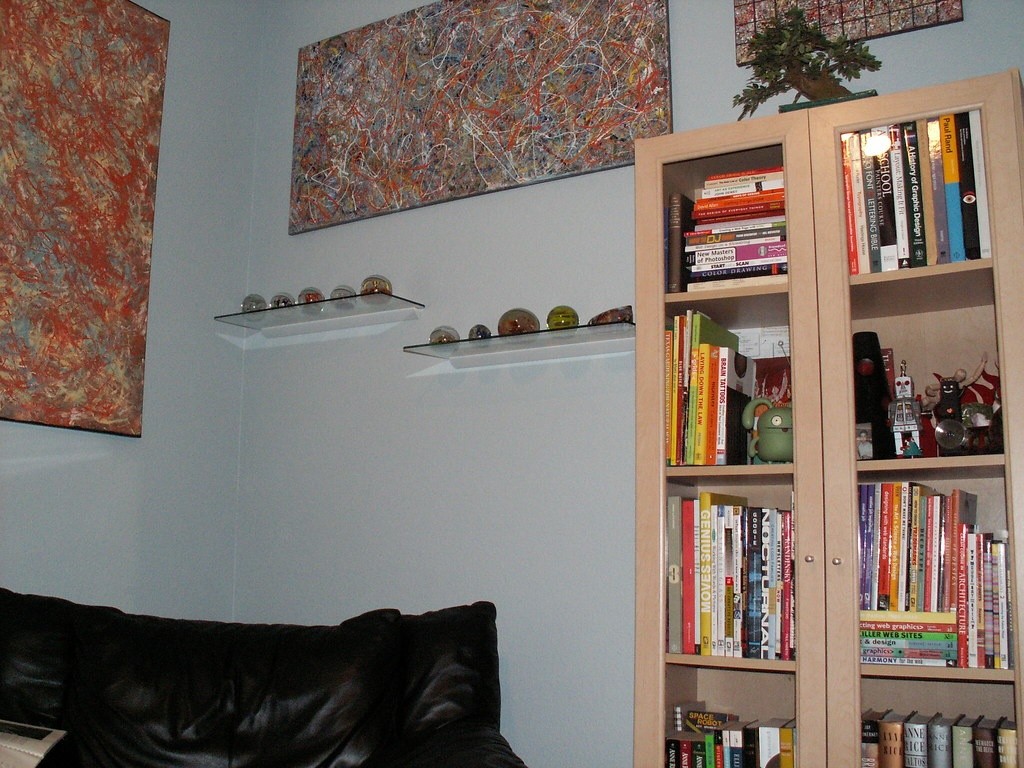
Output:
67;603;404;767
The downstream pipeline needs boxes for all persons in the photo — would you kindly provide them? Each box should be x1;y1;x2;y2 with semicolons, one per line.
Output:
858;430;873;459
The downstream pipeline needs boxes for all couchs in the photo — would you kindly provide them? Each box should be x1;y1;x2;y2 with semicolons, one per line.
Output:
0;585;538;768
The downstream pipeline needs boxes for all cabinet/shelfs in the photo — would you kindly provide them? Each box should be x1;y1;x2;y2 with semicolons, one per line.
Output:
633;68;1024;768
213;291;635;359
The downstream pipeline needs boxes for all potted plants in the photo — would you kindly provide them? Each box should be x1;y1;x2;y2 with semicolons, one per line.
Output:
733;3;882;122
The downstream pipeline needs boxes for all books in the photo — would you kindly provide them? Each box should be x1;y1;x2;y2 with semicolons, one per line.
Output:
664;700;1018;768
665;308;757;467
663;109;992;294
666;480;1015;670
